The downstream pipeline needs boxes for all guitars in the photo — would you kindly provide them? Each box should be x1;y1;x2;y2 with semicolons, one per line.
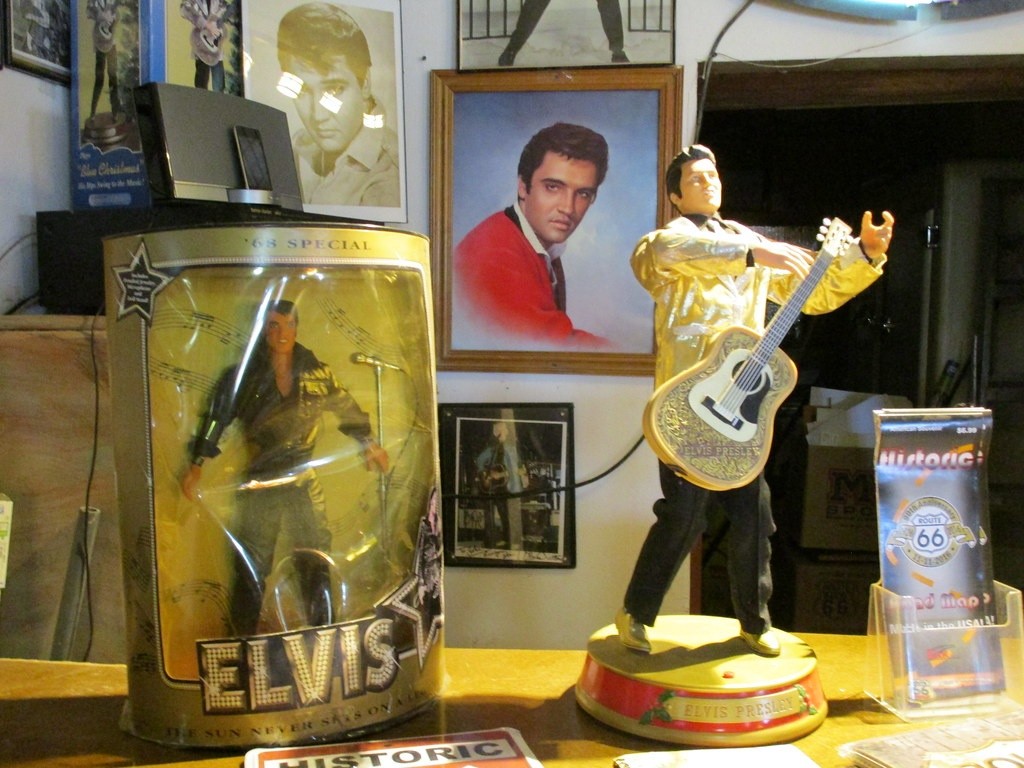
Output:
189;0;237;68
92;0;123;54
640;216;853;492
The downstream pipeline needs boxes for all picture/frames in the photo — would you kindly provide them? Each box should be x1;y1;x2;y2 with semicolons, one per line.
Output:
242;0;407;222
0;0;71;88
428;66;684;377
454;0;675;75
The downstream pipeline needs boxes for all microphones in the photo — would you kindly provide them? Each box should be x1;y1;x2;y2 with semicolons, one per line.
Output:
351;352;400;371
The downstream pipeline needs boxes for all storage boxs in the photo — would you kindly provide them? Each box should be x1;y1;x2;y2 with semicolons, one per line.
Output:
784;553;882;635
70;0;240;205
794;385;916;552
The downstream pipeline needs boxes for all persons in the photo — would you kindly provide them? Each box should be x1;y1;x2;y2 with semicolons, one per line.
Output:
179;0;232;90
614;146;895;655
278;2;400;207
20;0;60;59
495;0;629;66
182;300;387;636
454;123;609;349
474;423;527;548
86;0;119;121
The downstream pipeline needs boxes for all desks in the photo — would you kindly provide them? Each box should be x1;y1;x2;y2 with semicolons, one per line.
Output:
0;633;1024;768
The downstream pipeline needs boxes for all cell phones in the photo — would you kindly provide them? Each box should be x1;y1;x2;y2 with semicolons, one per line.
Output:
233;124;272;192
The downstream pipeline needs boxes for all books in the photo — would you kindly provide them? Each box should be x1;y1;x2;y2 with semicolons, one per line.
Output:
845;711;1023;768
614;744;820;768
